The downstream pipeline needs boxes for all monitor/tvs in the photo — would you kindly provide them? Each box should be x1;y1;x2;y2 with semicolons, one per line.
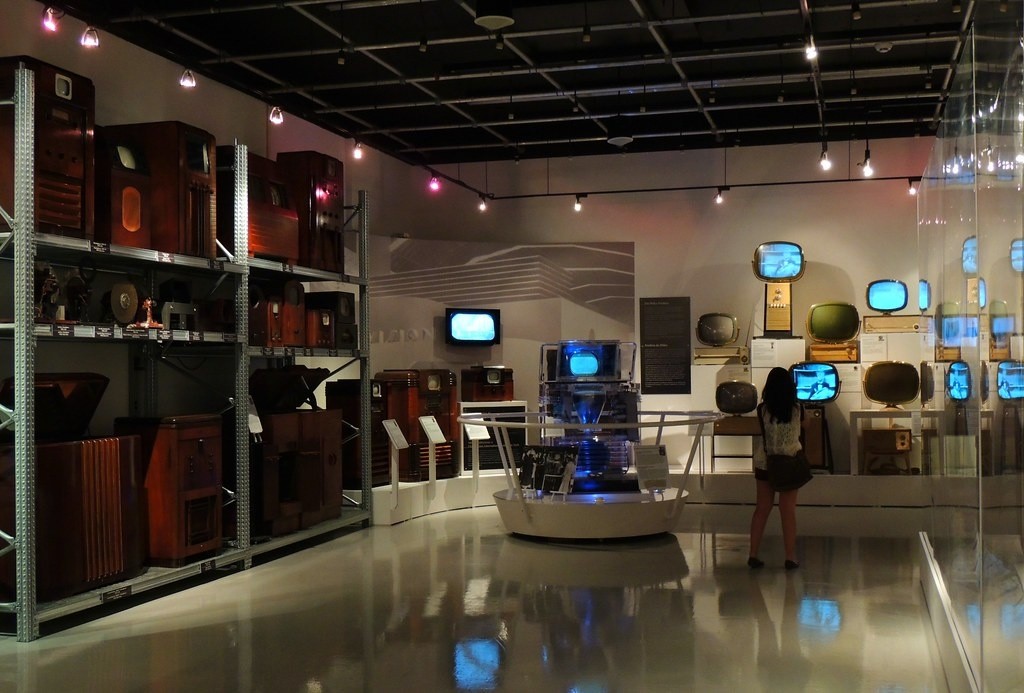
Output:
805;301;862;343
751;242;806;282
866;280;908;316
789;362;839;403
695;312;740;348
445;308;501;346
716;381;757;418
863;361;920;411
919;236;1024;404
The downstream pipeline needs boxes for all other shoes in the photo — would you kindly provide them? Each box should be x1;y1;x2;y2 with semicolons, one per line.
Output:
748;558;764;568
784;560;800;570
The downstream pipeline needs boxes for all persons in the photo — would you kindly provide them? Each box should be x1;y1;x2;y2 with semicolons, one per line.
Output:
743;366;815;569
808;370;829;399
998;380;1011;398
950;380;961;399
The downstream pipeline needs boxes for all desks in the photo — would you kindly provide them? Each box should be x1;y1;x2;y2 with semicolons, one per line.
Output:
850;410;921;475
919;409;995;476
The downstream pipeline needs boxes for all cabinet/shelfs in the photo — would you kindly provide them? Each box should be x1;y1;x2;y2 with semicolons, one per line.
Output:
690;333;920;475
0;68;375;642
922;333;1024;476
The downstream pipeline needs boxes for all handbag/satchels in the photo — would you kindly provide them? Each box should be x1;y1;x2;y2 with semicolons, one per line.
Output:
766;450;814;493
